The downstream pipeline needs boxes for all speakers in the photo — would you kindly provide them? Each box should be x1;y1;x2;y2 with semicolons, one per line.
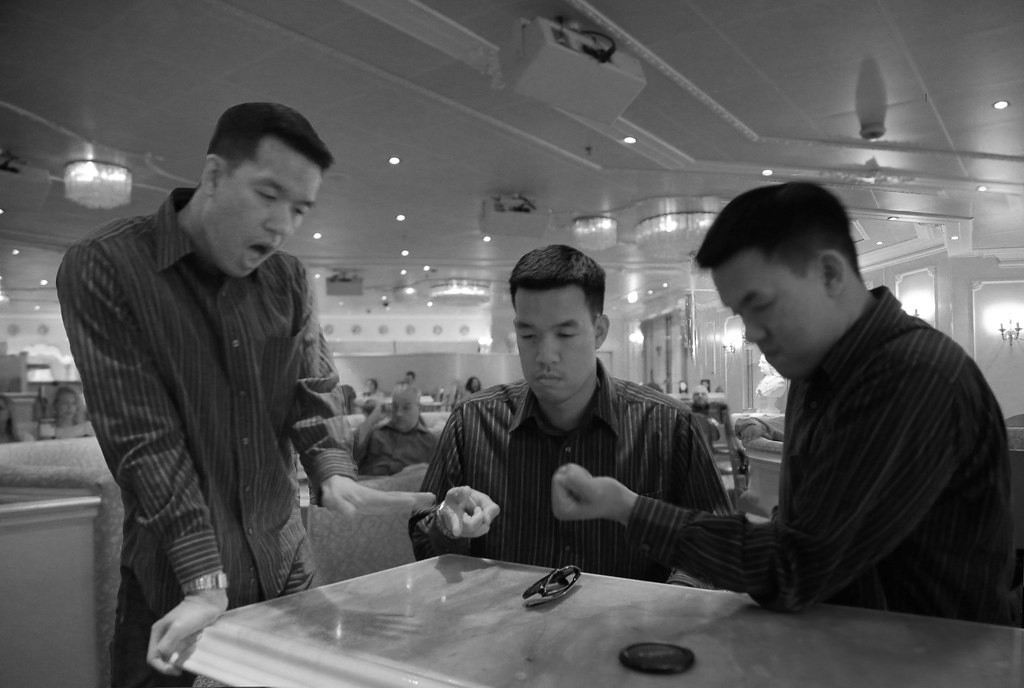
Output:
478;195;548;239
497;17;647;125
325;277;364;296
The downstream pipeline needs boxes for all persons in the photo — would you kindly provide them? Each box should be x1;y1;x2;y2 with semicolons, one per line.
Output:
52;387;83;429
551;181;1016;628
405;245;737;591
342;371;721;477
56;102;438;688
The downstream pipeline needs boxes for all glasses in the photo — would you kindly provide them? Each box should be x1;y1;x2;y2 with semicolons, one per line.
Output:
523;564;582;608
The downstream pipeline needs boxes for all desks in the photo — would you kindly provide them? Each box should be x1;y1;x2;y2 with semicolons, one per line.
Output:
162;553;1024;688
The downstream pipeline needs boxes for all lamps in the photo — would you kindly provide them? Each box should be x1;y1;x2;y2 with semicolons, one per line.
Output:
574;215;617;251
635;211;717;246
723;342;736;354
999;320;1023;346
62;159;133;209
431;283;490;296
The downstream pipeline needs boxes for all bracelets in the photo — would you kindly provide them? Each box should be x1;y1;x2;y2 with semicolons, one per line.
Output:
182;573;227;595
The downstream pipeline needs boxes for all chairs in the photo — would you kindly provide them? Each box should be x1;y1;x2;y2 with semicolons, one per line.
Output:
0;388;786;688
